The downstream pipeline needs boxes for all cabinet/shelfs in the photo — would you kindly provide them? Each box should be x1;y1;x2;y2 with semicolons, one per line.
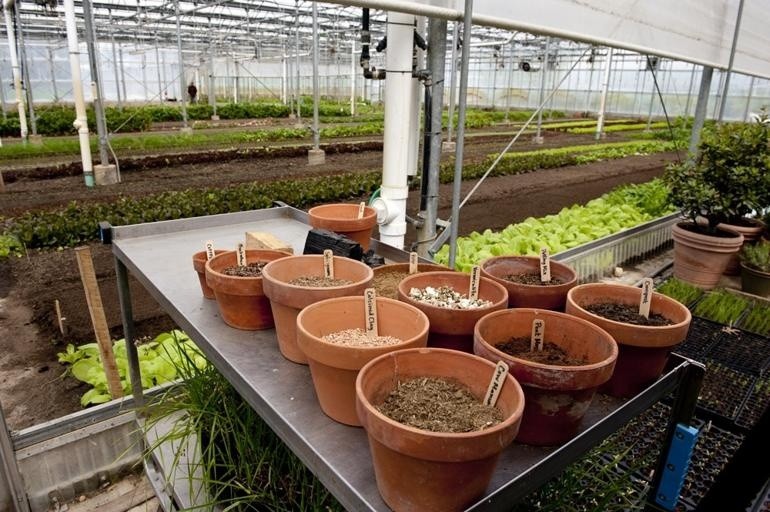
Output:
98;206;708;512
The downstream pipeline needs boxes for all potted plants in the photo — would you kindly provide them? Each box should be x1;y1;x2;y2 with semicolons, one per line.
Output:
113;332;245;512
666;102;770;298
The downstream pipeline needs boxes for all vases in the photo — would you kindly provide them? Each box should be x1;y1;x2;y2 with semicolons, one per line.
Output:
356;348;526;511
204;250;291;331
480;255;577;312
567;282;692;398
309;203;376;253
370;262;454;299
260;254;374;367
194;250;229;300
474;308;619;447
295;295;429;427
397;270;510;352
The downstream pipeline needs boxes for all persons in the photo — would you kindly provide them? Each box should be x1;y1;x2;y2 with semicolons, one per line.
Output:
188;82;198;102
